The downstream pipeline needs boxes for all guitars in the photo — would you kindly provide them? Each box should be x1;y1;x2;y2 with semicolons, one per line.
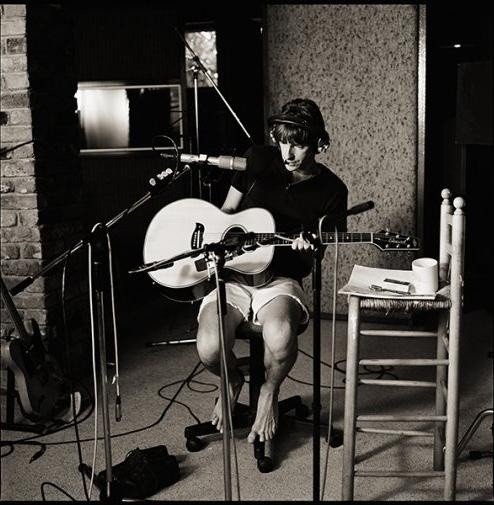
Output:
142;197;421;302
1;273;64;421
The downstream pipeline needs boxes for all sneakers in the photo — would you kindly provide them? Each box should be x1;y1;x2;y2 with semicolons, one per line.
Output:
122;454;180;499
97;445;170;485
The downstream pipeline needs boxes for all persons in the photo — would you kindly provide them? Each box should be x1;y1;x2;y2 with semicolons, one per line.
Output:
196;99;348;445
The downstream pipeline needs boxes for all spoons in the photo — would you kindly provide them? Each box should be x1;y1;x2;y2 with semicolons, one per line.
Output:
369;284;408;295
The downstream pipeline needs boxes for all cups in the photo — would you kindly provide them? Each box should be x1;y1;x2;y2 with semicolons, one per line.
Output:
411;258;438;294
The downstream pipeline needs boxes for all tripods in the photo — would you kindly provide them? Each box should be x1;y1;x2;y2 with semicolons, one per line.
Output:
8;164;190;501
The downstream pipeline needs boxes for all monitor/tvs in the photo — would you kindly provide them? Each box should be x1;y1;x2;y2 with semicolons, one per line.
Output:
74;84;184;153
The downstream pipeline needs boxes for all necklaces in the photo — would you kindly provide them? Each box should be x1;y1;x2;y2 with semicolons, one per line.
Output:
283;174;311;191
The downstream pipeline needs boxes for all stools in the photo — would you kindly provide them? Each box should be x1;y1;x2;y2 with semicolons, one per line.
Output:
187;304;344;474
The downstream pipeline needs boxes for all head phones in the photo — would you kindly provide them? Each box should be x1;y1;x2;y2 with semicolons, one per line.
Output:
266;118;330;155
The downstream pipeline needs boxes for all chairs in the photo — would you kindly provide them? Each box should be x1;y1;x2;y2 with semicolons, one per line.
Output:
338;188;466;501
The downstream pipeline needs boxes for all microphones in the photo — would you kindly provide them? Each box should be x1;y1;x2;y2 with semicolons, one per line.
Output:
180;153;247;171
276;143;289;164
130;259;174;273
